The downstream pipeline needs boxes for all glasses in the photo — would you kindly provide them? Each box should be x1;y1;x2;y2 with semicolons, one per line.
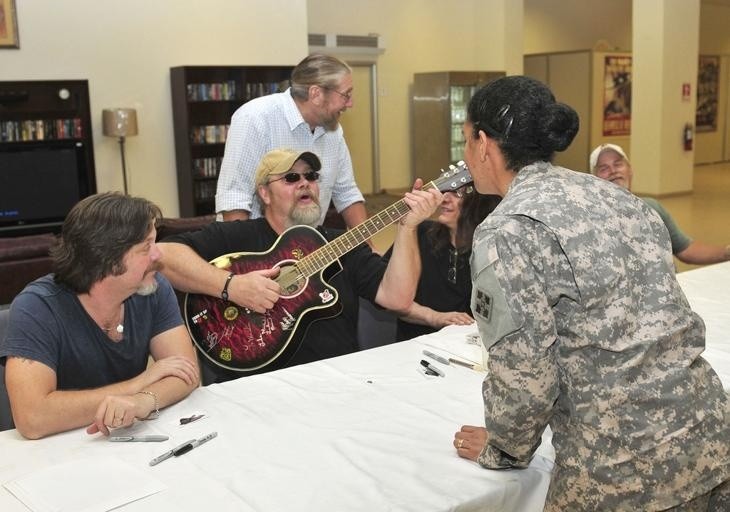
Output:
267;172;319;184
320;86;352;99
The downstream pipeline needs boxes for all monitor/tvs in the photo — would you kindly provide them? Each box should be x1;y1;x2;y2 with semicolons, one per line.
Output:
0;135;91;239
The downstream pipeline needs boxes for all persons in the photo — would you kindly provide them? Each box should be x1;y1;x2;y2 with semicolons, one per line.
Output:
589;142;730;266
382;184;504;342
0;190;201;439
214;52;381;255
454;74;730;511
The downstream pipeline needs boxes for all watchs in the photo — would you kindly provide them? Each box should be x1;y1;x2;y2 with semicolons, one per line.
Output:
155;149;448;383
221;271;237;303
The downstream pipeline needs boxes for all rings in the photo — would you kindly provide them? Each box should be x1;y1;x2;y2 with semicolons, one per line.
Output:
458;438;463;448
114;417;123;420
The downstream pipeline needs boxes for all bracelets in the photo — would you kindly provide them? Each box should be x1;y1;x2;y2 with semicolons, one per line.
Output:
134;390;160;421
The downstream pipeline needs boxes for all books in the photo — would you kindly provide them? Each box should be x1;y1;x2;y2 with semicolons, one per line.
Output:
192;157;223;202
454;88;469;150
188;80;284;100
1;116;84;141
189;125;230;142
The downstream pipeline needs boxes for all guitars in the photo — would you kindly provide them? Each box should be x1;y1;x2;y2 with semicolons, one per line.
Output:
184;160;475;377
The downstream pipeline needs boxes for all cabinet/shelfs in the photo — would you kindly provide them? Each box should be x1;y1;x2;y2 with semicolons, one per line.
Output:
0;78;93;144
169;64;295;217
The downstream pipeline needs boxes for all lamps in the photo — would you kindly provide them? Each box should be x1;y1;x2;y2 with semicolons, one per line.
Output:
100;106;139;194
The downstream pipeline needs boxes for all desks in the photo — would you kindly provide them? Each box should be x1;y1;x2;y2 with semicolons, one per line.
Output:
0;256;730;510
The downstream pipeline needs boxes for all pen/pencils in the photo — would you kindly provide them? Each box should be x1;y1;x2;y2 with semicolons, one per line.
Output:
108;434;169;442
420;360;445;377
448;357;475;370
174;431;218;456
149;438;197;467
422;349;450;365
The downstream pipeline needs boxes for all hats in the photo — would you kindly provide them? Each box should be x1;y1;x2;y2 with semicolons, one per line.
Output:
590;143;628;168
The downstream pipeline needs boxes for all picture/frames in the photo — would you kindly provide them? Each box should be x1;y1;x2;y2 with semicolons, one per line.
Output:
0;1;20;50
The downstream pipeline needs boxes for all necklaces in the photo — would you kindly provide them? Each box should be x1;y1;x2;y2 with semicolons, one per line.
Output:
98;319;125;341
